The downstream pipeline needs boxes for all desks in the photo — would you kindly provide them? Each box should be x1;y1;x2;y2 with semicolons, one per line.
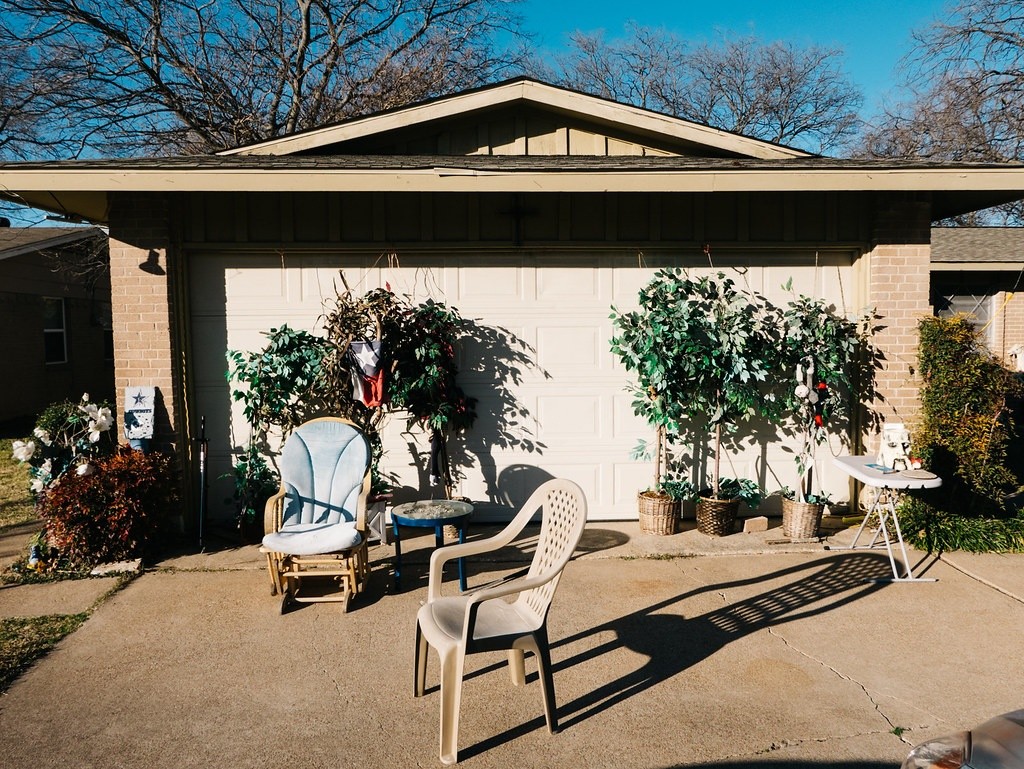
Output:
824;456;942;583
391;499;475;591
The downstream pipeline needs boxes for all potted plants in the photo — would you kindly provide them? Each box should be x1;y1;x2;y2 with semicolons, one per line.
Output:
609;266;868;538
215;282;479;545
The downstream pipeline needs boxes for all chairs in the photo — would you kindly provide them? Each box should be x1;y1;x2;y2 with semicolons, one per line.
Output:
412;478;587;766
262;418;372;616
